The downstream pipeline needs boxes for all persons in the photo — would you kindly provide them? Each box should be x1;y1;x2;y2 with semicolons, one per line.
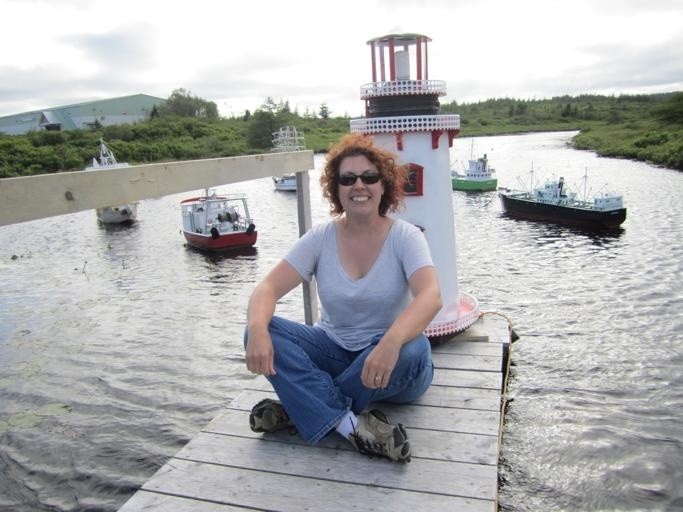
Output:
238;135;445;463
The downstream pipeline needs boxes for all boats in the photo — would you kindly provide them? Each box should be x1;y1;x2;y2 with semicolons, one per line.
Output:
179;186;257;252
84;144;137;225
498;159;626;232
272;126;306;192
451;140;498;192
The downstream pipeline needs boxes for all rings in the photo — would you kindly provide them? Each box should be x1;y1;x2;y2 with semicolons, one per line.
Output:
372;375;383;382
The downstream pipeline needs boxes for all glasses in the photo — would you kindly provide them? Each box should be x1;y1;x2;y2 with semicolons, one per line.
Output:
333;170;384;186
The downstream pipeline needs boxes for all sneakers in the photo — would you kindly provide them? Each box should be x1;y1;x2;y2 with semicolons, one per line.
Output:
349;409;411;465
248;397;295;435
